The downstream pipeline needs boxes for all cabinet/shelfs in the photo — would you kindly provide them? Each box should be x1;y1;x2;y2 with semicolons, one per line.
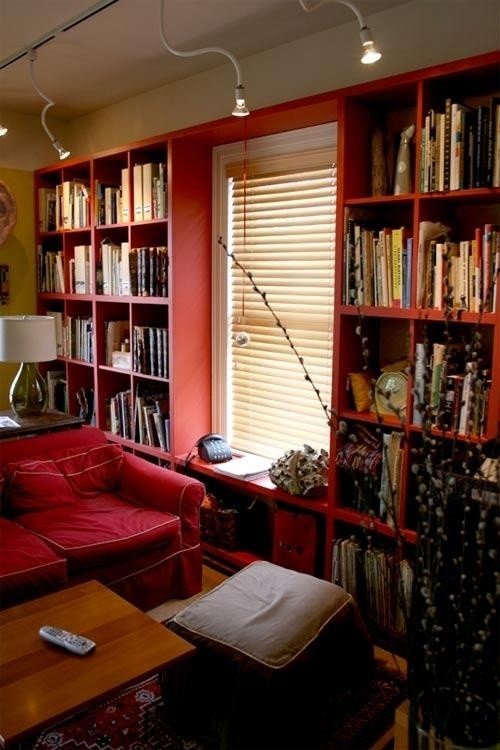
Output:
31;133;173;468
174;460;330;586
330;51;499;663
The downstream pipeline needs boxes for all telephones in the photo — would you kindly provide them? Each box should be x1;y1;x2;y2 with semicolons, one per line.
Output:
195;434;232;465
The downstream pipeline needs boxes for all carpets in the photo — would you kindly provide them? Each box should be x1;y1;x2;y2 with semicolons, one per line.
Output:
14;615;411;749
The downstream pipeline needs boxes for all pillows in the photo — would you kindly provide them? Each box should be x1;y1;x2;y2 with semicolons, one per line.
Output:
8;443;124;510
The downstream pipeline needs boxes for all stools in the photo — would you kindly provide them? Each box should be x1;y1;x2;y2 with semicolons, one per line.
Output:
168;561;374;736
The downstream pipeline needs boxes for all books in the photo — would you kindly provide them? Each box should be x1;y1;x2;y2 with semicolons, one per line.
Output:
337;422;405;529
42;369;170;452
342;204;499;313
411;338;489;438
40;311;168;377
33;161;169;233
420;95;499;192
36;238;167;296
330;538;414;635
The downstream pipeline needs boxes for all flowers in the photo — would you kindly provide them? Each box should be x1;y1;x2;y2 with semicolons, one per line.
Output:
216;234;500;750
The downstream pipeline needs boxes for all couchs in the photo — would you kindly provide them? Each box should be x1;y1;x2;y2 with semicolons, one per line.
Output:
0;425;204;612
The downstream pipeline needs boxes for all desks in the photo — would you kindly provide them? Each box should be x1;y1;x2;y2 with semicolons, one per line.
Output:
1;578;197;748
0;409;84;436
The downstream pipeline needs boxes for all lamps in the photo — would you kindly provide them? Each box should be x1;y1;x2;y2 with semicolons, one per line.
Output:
158;1;248;118
0;316;57;416
300;0;381;65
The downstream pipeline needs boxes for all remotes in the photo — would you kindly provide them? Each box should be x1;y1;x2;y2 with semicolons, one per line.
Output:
39;625;96;655
